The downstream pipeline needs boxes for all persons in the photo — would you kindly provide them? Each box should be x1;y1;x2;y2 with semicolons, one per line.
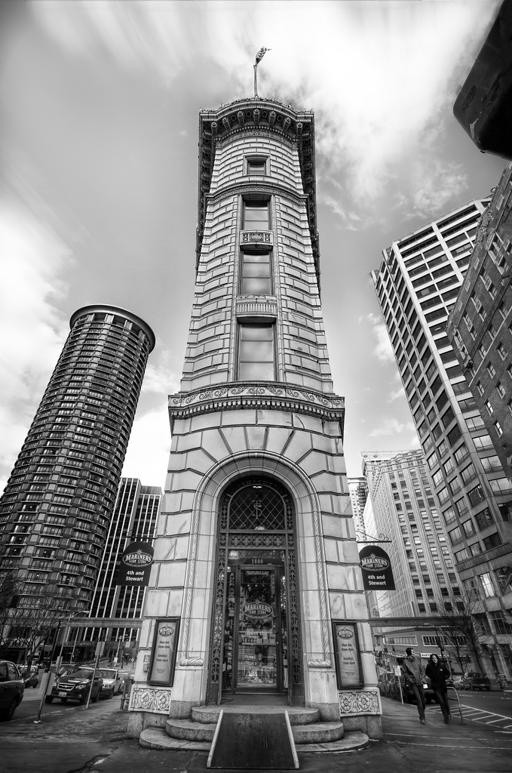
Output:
402;648;427;725
425;654;451;724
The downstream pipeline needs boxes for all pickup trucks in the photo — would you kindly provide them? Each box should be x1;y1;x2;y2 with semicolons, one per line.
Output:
461;671;492;692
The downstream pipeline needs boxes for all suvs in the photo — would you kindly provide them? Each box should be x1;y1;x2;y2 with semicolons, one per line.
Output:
0;660;27;720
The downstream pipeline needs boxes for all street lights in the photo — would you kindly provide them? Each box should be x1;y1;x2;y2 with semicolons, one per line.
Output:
56;610;90;674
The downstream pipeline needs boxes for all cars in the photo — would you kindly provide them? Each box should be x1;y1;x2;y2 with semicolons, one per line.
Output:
452;674;464;690
15;659;137;707
379;672;436;704
